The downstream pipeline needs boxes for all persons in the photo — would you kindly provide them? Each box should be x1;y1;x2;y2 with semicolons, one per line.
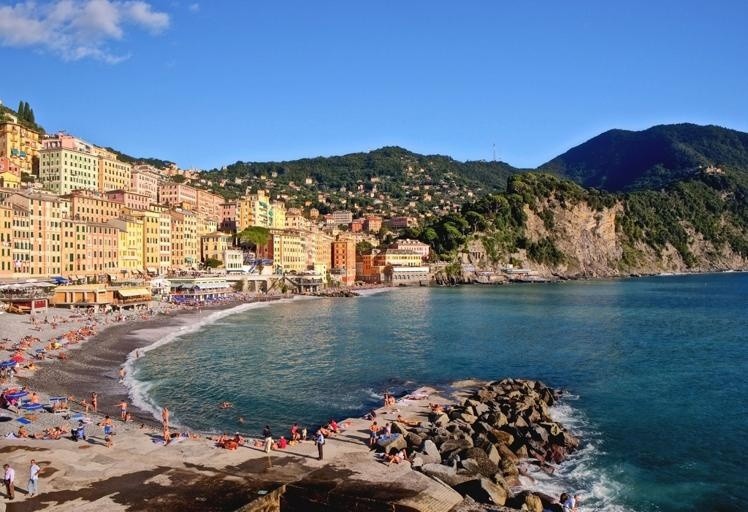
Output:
1;463;16;500
378;451;402;466
562;494;580;512
25;458;41;497
314;428;326;461
